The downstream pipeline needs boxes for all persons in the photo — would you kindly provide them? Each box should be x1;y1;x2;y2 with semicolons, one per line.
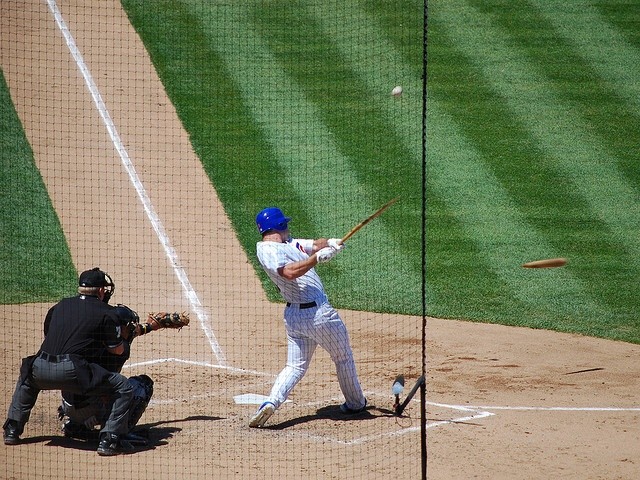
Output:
247;207;367;429
2;266;137;456
59;273;169;444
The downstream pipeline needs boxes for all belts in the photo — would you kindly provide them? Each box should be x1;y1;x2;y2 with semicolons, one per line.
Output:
77;401;91;408
40;351;84;363
287;301;317;309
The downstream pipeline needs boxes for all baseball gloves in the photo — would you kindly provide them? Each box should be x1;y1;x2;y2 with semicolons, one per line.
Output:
147;312;189;331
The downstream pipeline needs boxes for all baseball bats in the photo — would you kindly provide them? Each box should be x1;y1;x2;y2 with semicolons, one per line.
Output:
522;259;565;268
339;200;395;245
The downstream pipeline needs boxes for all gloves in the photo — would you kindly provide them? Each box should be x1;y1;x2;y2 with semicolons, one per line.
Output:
78;406;98;430
315;247;336;263
327;238;346;254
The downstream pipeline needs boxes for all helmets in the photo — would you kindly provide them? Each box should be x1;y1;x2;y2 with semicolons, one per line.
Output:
79;268;115;303
256;208;292;234
114;304;139;344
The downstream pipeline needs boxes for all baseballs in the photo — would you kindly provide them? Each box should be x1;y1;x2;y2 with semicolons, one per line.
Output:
392;86;402;96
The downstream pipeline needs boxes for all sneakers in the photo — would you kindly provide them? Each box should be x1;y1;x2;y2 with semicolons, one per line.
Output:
249;402;276;428
340;398;366;418
97;437;135;455
122;433;148;446
3;423;19;444
62;420;100;439
